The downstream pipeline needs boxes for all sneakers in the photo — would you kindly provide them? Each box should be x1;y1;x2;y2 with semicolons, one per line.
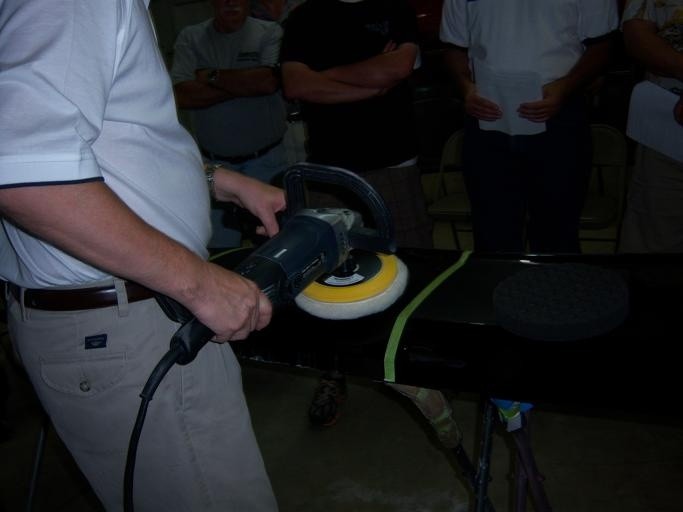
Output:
308;370;348;430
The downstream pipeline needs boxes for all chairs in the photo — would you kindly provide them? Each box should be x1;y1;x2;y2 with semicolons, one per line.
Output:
570;115;628;252
425;130;478;250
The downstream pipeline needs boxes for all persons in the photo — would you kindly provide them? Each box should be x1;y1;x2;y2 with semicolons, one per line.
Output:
621;0;683;252
278;0;434;425
0;0;287;512
440;0;620;256
170;0;305;245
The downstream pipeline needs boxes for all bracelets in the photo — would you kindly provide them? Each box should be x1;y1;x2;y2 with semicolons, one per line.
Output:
205;164;220;203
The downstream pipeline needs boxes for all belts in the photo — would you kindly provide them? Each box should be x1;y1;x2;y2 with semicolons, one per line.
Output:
199;134;286;164
0;282;157;312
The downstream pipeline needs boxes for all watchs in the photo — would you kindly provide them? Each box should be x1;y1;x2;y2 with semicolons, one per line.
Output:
208;69;219;86
